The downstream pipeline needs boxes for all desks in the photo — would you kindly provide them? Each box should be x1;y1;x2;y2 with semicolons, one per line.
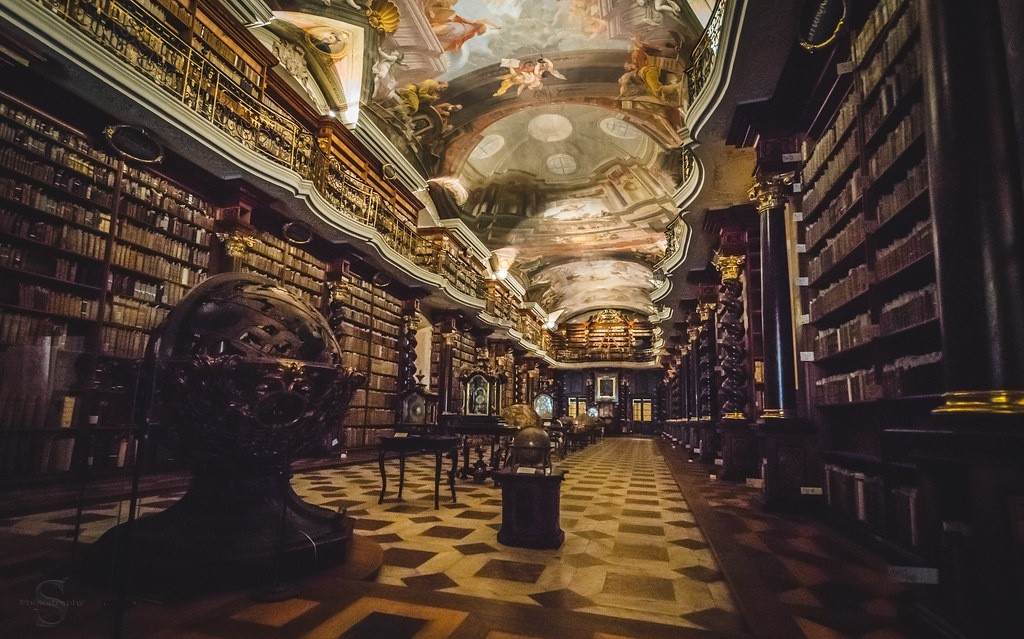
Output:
375;435;458;510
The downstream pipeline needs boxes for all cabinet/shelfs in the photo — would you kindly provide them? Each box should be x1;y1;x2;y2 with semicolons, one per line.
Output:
657;0;1024;639
484;278;557;360
417;227;487;297
560;309;654;362
36;0;269;152
0;63;425;517
